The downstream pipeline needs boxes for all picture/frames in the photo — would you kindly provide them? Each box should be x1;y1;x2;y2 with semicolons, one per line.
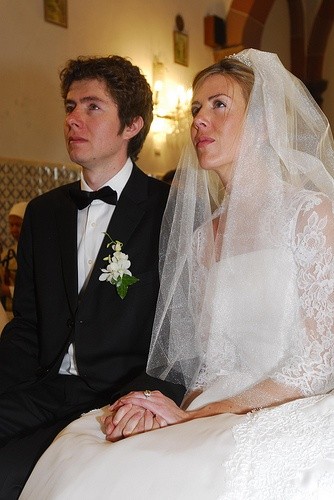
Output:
44;0;68;29
173;30;189;66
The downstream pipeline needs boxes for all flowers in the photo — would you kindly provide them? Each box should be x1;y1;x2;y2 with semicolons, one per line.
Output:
99;231;140;299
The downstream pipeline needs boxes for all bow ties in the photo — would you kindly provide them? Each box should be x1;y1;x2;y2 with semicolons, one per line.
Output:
76;185;118;211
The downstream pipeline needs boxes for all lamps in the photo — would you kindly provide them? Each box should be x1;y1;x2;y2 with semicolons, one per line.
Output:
151;55;192;120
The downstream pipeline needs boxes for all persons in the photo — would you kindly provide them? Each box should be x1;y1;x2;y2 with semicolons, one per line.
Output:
0;55;204;500
17;48;334;500
0;201;29;313
162;169;186;190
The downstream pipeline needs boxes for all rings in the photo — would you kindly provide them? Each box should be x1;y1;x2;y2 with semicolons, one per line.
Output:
144;390;151;398
